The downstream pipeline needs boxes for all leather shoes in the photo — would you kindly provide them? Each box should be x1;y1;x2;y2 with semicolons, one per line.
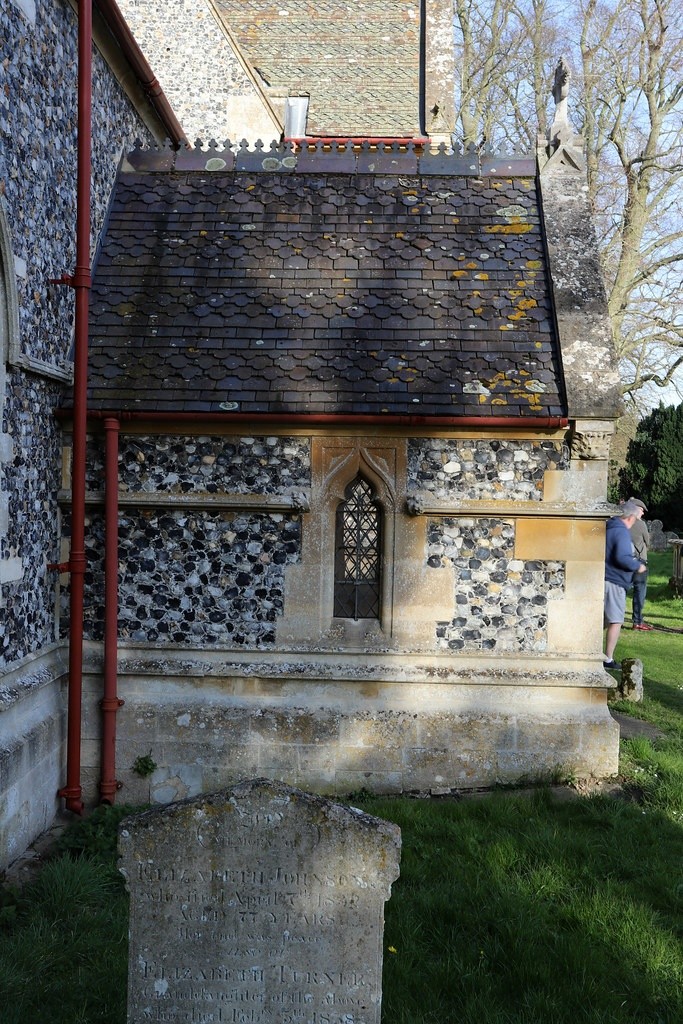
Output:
631;622;653;631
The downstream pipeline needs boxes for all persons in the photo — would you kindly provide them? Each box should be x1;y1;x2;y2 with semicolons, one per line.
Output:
603;502;646;671
628;497;653;631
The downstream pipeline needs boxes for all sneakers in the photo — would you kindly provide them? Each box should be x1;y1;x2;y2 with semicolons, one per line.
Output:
604;659;622;671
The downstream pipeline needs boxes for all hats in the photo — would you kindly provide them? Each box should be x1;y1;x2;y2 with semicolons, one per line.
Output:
628;498;649;511
